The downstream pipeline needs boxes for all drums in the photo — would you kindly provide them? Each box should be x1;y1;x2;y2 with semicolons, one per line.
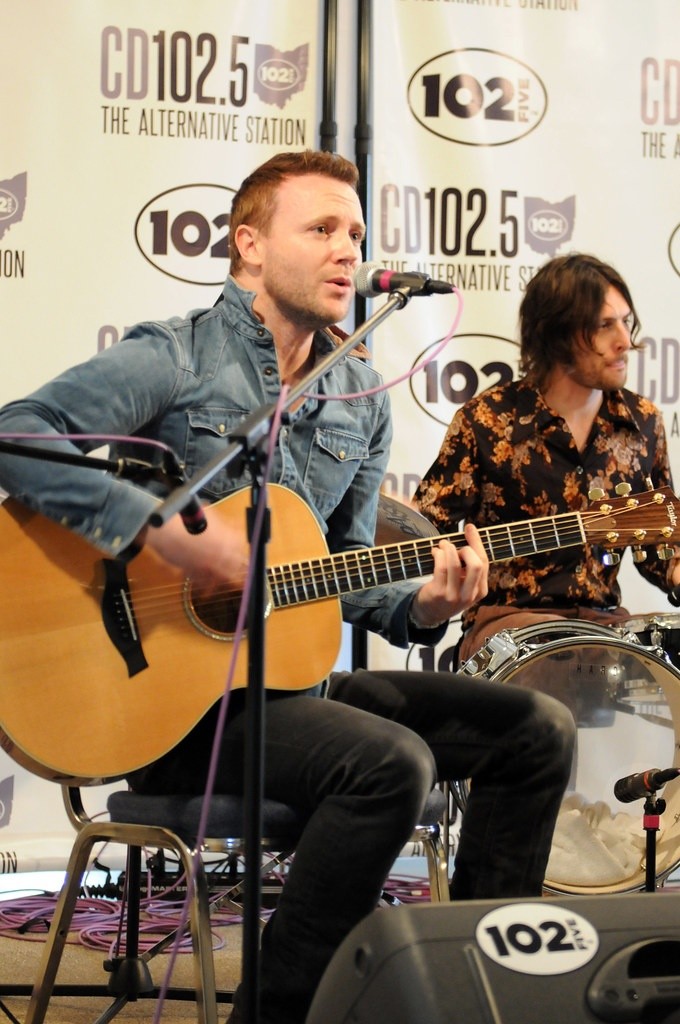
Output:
628;611;680;668
447;618;680;895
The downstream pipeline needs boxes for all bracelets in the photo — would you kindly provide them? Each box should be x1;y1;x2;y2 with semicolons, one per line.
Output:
409;606;448;628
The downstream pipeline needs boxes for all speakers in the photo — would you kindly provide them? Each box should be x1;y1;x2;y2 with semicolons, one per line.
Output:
305;892;680;1024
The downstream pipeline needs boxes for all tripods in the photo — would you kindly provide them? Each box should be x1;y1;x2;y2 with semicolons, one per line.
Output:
0;846;237;1024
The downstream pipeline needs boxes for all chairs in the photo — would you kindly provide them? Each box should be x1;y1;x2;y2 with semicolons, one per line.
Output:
26;784;449;1024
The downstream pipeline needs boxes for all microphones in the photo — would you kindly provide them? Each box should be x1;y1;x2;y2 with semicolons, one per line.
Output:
614;768;680;803
354;261;456;299
162;449;207;534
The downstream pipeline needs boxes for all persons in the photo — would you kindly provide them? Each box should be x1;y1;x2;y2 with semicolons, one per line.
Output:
0;150;575;1024
414;253;680;672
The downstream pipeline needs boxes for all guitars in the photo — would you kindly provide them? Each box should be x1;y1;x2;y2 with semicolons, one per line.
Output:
0;482;680;791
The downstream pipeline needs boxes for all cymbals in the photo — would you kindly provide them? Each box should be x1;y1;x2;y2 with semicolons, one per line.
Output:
375;491;439;543
